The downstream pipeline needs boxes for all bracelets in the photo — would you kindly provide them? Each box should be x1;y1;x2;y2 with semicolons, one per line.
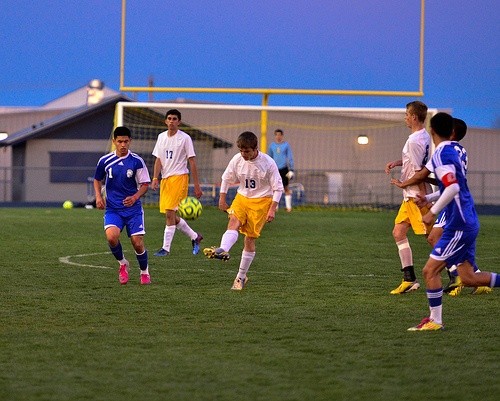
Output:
133;196;137;201
153;178;158;180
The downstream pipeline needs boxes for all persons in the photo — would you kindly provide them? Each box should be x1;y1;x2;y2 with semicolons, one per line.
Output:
151;109;203;256
93;127;152;285
203;131;286;290
385;101;454;294
267;129;294;211
391;113;500;330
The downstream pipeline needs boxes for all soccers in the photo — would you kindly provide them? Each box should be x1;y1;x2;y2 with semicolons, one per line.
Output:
63;200;73;209
178;196;202;220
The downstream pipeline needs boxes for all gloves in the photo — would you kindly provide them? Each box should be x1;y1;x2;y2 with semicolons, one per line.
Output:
286;170;295;179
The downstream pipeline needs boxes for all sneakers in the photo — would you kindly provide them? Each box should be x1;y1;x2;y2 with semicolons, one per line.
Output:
230;277;249;291
203;246;230;262
118;260;130;285
390;279;420;295
153;248;170;257
192;233;203;256
443;280;456;294
448;277;464;298
407;318;444;332
140;272;150;285
468;286;493;295
